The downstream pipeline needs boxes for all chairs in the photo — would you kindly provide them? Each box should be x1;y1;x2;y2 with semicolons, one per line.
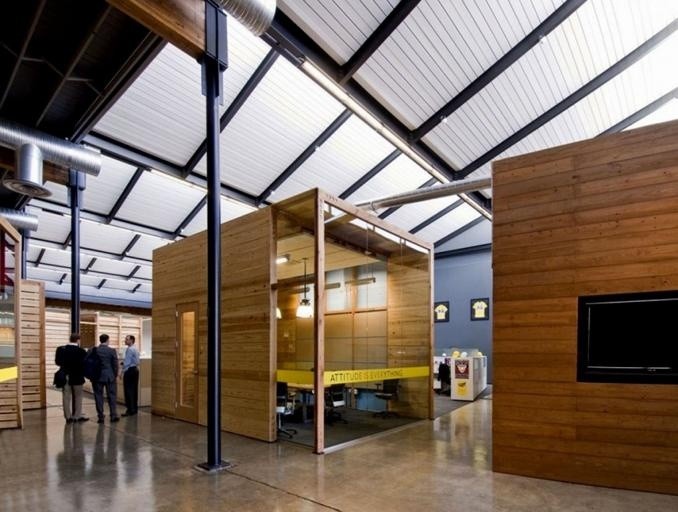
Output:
324;384;349;426
373;379;400;419
275;382;297;439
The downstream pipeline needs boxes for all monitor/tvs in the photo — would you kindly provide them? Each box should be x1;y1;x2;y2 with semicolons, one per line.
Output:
576;289;678;385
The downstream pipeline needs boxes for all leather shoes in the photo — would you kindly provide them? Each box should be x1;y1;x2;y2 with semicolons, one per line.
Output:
97;419;104;424
121;411;138;416
66;417;90;424
110;417;120;423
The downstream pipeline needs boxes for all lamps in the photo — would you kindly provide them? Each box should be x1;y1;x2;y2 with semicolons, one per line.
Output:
276;254;375;318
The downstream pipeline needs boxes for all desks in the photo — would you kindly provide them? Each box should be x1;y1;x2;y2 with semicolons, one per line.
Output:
288;383;354;424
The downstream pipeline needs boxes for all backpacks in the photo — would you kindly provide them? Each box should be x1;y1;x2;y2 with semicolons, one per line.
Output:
84;347;102;379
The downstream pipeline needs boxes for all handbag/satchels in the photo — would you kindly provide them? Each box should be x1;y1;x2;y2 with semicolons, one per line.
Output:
53;369;67;388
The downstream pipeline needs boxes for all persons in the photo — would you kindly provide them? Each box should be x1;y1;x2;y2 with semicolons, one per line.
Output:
118;335;140;416
82;333;120;423
91;423;118;504
121;417;139;484
56;423;87;508
55;333;90;423
